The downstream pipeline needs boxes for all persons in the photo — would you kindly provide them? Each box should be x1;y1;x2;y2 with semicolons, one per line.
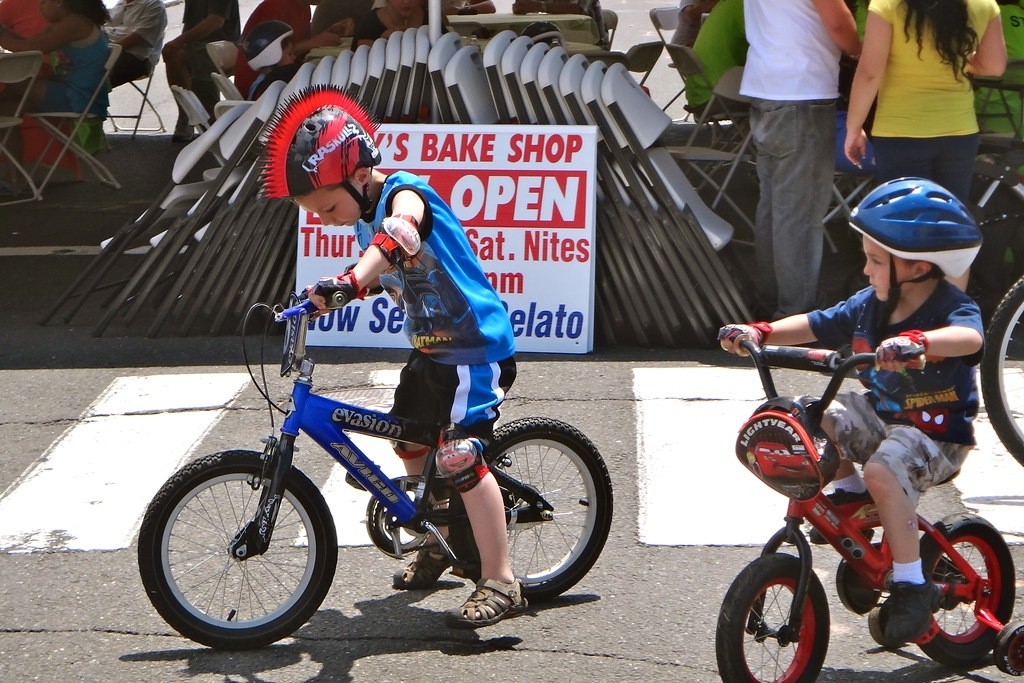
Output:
718;177;986;651
234;0;356;97
161;0;240;142
311;1;610;53
738;0;863;323
843;0;1008;206
670;0;1024;172
0;0;112;195
257;83;529;630
101;0;167;89
243;20;296;101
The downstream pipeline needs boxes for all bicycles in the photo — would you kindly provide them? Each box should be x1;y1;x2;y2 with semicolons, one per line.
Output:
715;332;1024;683
980;273;1024;468
137;290;613;653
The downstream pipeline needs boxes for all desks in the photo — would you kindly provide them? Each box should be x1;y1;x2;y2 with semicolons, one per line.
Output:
459;39;604;58
446;14;600;44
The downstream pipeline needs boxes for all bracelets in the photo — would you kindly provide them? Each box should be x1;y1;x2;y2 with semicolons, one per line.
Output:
849;55;860;60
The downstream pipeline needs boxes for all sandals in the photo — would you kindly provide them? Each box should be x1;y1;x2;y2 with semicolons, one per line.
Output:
393;536;454;589
445;578;528;629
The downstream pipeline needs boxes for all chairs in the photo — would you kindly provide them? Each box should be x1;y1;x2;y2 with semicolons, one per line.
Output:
0;0;1024;347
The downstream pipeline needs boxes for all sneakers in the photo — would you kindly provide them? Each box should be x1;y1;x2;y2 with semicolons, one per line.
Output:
884;572;946;645
171;119;194;140
809;488;870;544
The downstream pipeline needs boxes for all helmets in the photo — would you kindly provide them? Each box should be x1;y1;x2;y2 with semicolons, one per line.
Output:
850;176;983;277
257;83;383;200
243;20;294;70
